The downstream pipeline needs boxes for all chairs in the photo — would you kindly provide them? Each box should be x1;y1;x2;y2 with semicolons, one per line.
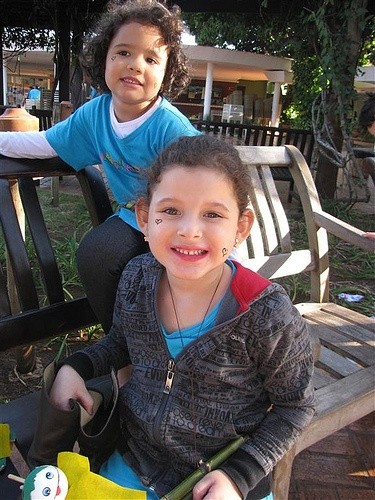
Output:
0;157;115;458
0;104;53;132
228;145;375;500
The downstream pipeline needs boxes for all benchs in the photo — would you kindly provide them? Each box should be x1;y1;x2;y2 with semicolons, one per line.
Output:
189;119;314;208
